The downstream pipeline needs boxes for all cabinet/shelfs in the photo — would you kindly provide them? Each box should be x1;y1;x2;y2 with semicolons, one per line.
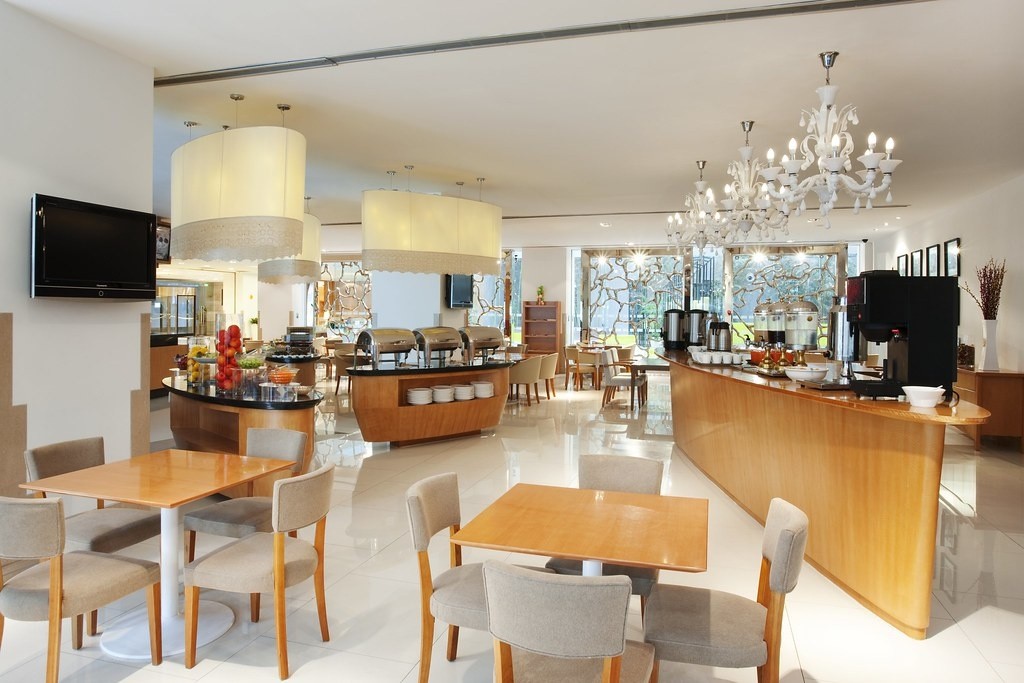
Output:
520;299;561;372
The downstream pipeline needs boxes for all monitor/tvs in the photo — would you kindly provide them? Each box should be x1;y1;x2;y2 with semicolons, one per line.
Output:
446;273;474;309
29;192;157;302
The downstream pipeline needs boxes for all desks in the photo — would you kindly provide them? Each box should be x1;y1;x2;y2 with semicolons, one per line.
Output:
486;353;548;402
162;374;323;498
631;358;673;411
340;352;373;365
946;369;1024;450
450;483;711;575
17;447;297;660
577;349;611;390
343;359;515;451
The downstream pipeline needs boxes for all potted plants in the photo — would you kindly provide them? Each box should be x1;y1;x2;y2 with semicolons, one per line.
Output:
252;316;259;341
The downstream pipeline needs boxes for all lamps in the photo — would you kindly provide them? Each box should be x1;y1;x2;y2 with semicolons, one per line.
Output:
170;125;307;261
361;190;504;273
259;213;322;284
664;45;907;252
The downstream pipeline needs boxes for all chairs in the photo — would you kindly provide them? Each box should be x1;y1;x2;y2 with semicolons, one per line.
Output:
487;559;658;683
489;342;648;411
544;456;666;627
183;428;312;621
641;497;810;683
27;431;164;634
178;462;339;680
406;471;554;683
319;331;377;395
1;493;168;683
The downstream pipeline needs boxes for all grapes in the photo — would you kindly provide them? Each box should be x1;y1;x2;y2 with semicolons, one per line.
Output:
238;358;264;369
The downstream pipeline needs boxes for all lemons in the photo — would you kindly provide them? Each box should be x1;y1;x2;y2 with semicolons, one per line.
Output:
186;345;210;382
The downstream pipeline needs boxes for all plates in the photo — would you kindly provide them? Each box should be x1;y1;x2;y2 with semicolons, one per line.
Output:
430;385;455;403
193;356;216;363
470;381;494;399
451;384;474;400
406;387;433;405
259;382;300;388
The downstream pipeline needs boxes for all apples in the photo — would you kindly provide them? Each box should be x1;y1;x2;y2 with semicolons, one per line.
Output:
214;325;241;390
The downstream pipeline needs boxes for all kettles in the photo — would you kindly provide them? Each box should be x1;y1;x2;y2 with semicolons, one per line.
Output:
708;322;731;351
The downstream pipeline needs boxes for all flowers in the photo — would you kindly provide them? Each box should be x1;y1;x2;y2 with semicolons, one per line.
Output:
958;257;1007;320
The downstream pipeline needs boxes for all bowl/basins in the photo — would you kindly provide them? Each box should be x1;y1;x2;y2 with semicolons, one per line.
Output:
902;386;945;408
784;367;829;381
266;368;300;384
293;385;312;396
174;356;187;369
233;354;266;369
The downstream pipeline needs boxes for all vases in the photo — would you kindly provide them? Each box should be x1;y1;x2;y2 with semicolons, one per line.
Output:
979;318;998;370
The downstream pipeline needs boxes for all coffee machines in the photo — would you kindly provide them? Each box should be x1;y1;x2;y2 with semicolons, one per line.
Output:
846;270;959;401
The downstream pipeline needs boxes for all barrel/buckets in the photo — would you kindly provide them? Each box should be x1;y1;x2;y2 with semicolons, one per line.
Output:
660;309;709;351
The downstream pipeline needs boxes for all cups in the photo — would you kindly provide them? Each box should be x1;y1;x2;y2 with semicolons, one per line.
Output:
214;313;244;393
687;346;743;364
187;336;210;386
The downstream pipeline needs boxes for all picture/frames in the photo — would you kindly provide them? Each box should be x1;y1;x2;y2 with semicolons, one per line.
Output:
943;237;960;277
896;254;908;277
926;243;940;276
911;248;923;275
